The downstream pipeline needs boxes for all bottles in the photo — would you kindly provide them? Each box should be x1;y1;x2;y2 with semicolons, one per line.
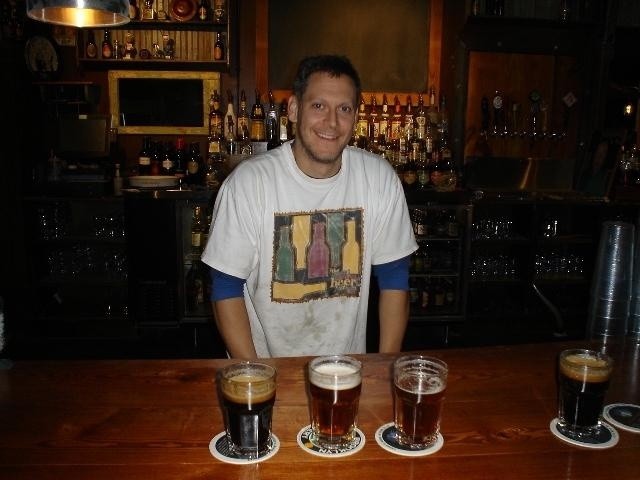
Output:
113;89;455;312
214;32;224;60
86;29;120;59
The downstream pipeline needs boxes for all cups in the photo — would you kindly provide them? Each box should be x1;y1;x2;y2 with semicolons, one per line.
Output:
37;212;128;281
469;214;640;438
217;355;448;458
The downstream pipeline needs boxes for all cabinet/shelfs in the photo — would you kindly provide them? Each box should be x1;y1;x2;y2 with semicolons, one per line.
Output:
0;0;238;71
1;178;640;347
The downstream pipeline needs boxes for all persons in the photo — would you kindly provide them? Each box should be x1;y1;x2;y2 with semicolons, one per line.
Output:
199;54;421;361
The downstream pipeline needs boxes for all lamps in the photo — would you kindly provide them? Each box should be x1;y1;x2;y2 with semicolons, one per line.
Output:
26;0;132;28
